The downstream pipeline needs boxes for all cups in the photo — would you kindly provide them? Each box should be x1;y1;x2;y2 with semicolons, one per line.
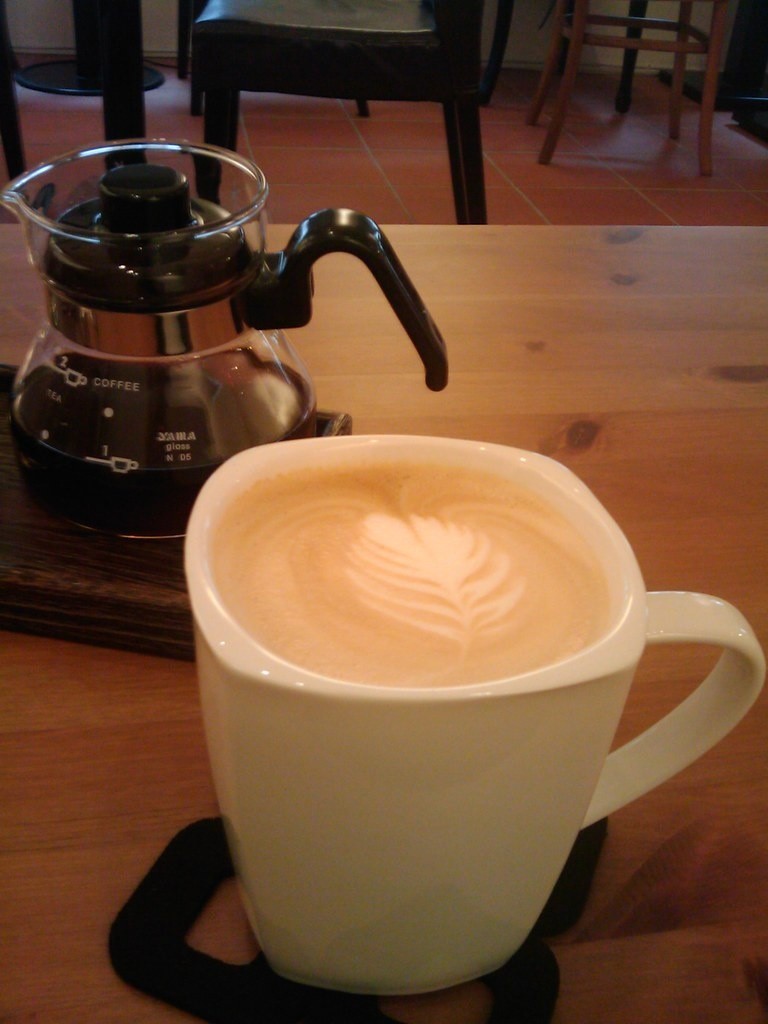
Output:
184;434;766;996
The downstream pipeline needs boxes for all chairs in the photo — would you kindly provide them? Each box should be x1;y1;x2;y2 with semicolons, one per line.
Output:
192;1;485;225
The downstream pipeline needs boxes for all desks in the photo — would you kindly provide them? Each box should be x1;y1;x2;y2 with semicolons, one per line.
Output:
0;219;768;1024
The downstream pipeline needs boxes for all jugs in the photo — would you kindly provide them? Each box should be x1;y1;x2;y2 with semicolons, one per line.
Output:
0;139;448;540
